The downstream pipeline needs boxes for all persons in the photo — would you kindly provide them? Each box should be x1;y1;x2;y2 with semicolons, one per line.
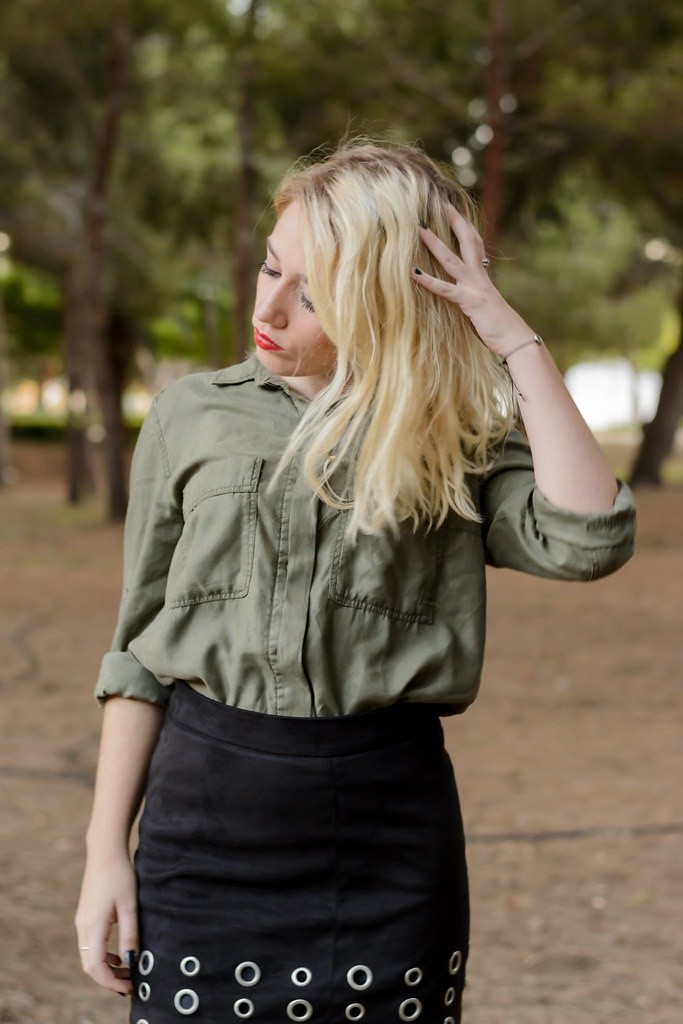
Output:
68;136;639;1024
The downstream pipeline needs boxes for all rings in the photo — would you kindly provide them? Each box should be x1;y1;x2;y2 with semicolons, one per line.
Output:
482;256;490;271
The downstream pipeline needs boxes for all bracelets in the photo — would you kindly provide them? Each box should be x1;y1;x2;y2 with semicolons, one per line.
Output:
500;331;543;366
